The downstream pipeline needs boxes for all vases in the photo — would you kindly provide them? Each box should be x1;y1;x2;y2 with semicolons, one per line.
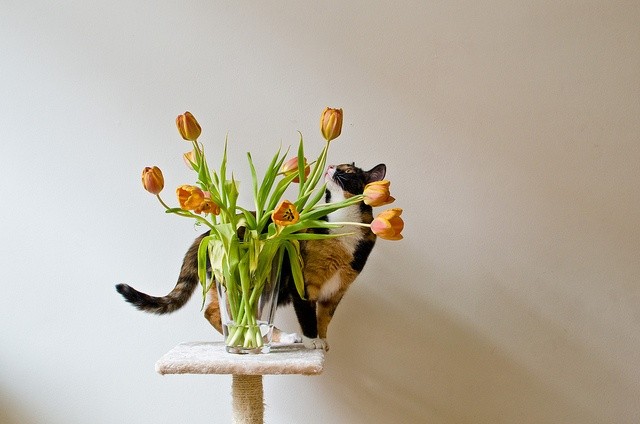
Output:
214;240;280;352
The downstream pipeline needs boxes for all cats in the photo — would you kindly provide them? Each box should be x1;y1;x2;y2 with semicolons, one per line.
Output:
115;162;386;351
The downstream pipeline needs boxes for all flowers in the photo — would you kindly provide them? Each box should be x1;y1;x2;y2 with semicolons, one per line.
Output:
141;108;404;351
